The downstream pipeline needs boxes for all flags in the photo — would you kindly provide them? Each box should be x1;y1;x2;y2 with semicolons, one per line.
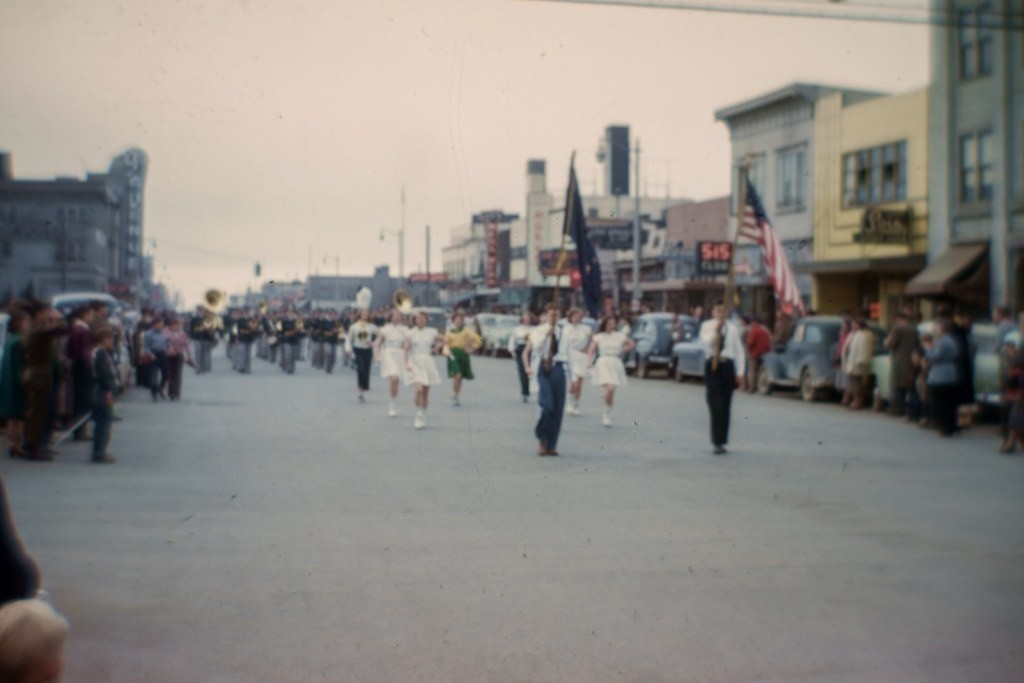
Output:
562;167;603;320
737;167;808;319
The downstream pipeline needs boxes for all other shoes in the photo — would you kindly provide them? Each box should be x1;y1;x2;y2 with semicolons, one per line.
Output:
739;378;1024;453
522;396;529;405
358;394;366;405
0;379;181;465
537;438;559;458
712;444;727;455
450;396;460;407
196;351;356;375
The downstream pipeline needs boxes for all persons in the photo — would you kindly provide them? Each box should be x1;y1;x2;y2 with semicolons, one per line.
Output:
507;311;538;404
0;597;71;683
402;310;448;428
556;306;596;416
442;312;482;407
342;307;383;403
88;327;116;463
164;318;195;401
527;302;570;457
0;481;43;607
593;292;815;394
697;298;748;456
583;314;636;427
831;305;1024;454
45;282;394;391
373;309;410;418
64;305;99;439
143;318;170;400
7;312;30;458
0;291;16;363
29;309;73;462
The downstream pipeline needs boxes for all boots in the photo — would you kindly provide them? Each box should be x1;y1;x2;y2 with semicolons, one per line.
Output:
414;406;428;428
566;393;580;416
604;405;616;428
388;395;398;418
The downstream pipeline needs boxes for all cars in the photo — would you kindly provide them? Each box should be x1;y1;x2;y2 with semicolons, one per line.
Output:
669;315;767;392
760;315;888;405
621;312;705;378
554;315;605;364
395;308;523;358
50;289;130;391
861;318;1020;420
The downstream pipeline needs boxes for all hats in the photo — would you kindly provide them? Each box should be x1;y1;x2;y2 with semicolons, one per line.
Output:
355;283;373;310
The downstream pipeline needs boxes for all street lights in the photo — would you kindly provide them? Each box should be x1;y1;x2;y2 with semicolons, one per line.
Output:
596;134;642;311
